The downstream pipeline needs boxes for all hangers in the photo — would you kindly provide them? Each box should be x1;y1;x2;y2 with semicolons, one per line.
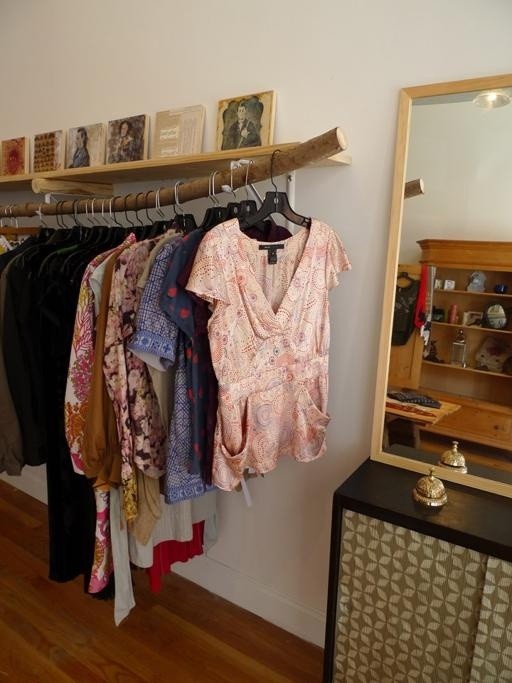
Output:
0;148;340;280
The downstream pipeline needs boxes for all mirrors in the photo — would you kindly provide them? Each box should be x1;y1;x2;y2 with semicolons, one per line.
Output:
371;73;512;500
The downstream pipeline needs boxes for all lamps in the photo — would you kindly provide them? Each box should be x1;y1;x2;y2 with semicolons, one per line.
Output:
476;90;510;110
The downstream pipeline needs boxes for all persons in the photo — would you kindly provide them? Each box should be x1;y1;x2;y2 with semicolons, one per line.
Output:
221;103;259;149
67;126;90;167
108;120;135;164
3;138;22;174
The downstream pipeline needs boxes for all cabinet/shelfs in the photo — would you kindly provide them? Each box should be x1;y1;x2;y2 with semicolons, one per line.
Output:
320;455;512;683
401;236;512;451
